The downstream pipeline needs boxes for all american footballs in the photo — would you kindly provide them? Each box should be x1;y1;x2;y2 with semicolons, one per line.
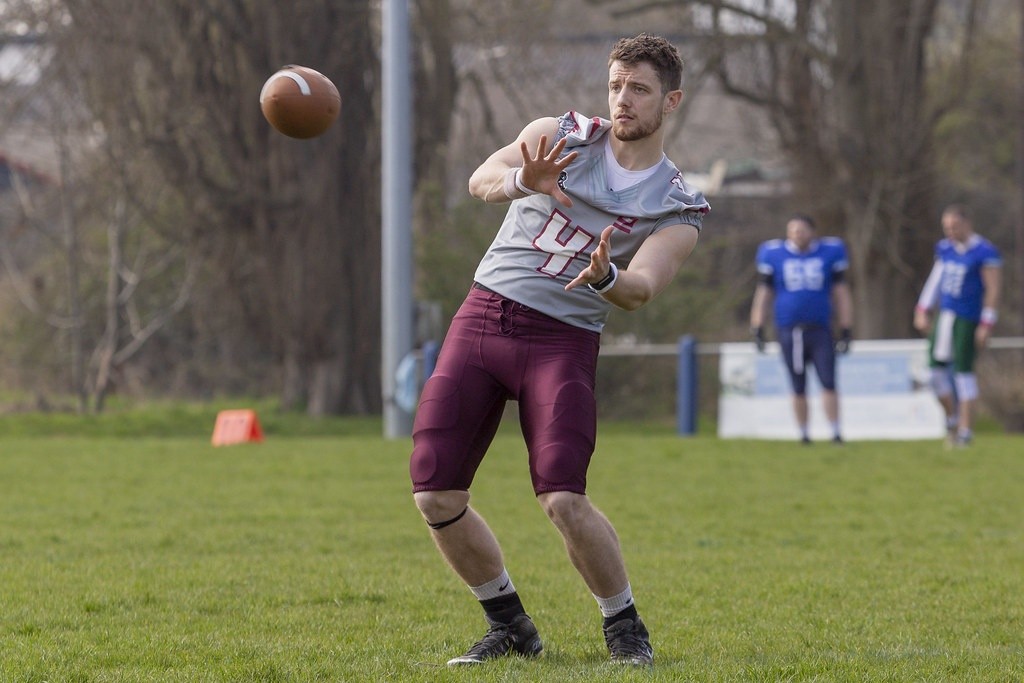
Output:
260;63;339;138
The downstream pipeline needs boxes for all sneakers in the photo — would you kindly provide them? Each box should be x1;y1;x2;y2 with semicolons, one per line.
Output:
602;618;656;673
447;612;548;669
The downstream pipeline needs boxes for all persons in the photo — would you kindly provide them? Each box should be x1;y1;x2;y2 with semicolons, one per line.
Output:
749;210;853;443
913;199;1001;446
407;34;710;670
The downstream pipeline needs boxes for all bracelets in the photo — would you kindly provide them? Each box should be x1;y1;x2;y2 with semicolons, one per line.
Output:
587;262;618;294
504;167;539;200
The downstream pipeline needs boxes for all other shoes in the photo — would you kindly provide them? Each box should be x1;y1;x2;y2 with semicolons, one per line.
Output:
943;427;973;446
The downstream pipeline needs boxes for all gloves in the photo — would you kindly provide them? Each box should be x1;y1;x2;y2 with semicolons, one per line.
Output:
752;327;766;351
833;328;853;353
